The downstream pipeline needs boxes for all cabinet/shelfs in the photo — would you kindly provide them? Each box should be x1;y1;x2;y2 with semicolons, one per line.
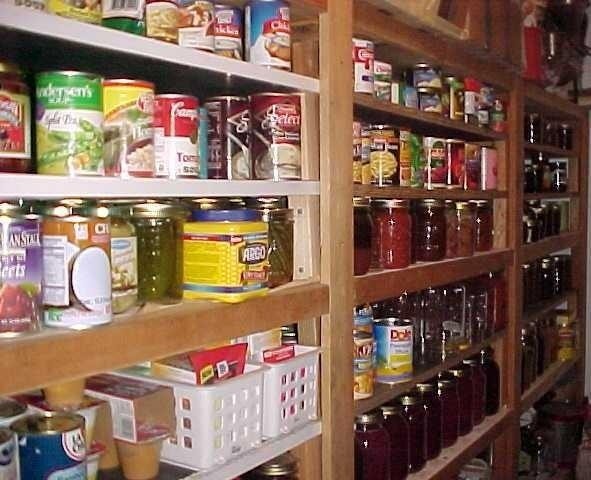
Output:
0;0;330;479
352;1;517;480
519;76;590;480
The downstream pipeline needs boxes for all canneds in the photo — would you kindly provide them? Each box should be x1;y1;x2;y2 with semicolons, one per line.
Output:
0;195;295;332
522;112;576;397
253;454;299;480
353;303;501;480
0;398;88;480
1;0;301;183
352;36;507;276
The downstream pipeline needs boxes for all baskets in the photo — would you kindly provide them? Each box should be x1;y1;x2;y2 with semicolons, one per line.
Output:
107;360;272;473
262;344;323;440
